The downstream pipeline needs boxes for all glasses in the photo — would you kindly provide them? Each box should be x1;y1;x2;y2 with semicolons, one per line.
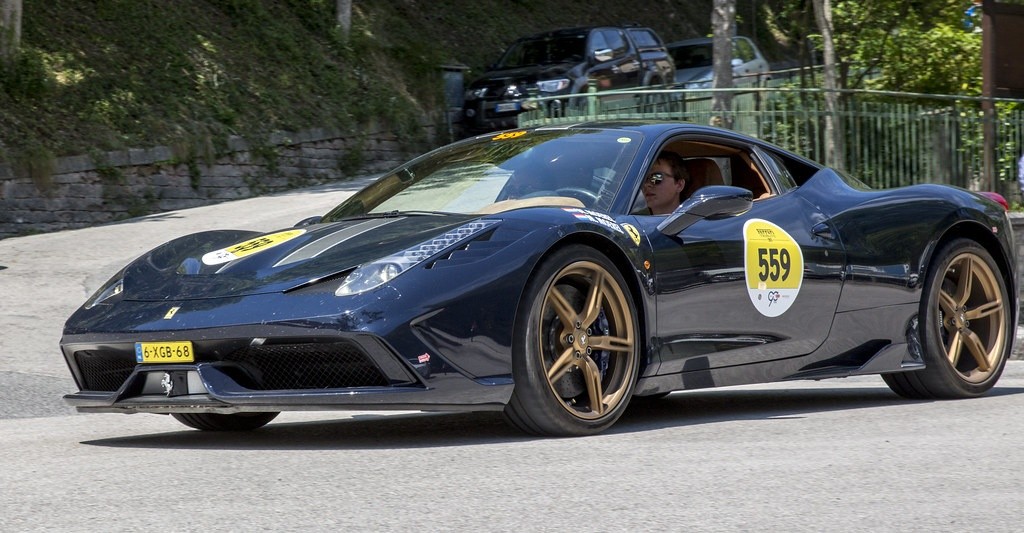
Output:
648;171;675;185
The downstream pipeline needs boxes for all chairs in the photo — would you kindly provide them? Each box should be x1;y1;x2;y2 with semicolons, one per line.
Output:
680;158;724;202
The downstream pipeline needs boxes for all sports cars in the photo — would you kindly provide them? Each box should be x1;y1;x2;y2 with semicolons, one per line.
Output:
58;120;1022;440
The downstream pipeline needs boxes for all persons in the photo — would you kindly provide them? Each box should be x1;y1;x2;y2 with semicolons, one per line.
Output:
626;152;690;216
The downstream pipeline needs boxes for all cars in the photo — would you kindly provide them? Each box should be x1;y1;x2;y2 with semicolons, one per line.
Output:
660;36;770;107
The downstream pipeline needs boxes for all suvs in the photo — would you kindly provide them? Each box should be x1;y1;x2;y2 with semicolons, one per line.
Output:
460;21;677;132
